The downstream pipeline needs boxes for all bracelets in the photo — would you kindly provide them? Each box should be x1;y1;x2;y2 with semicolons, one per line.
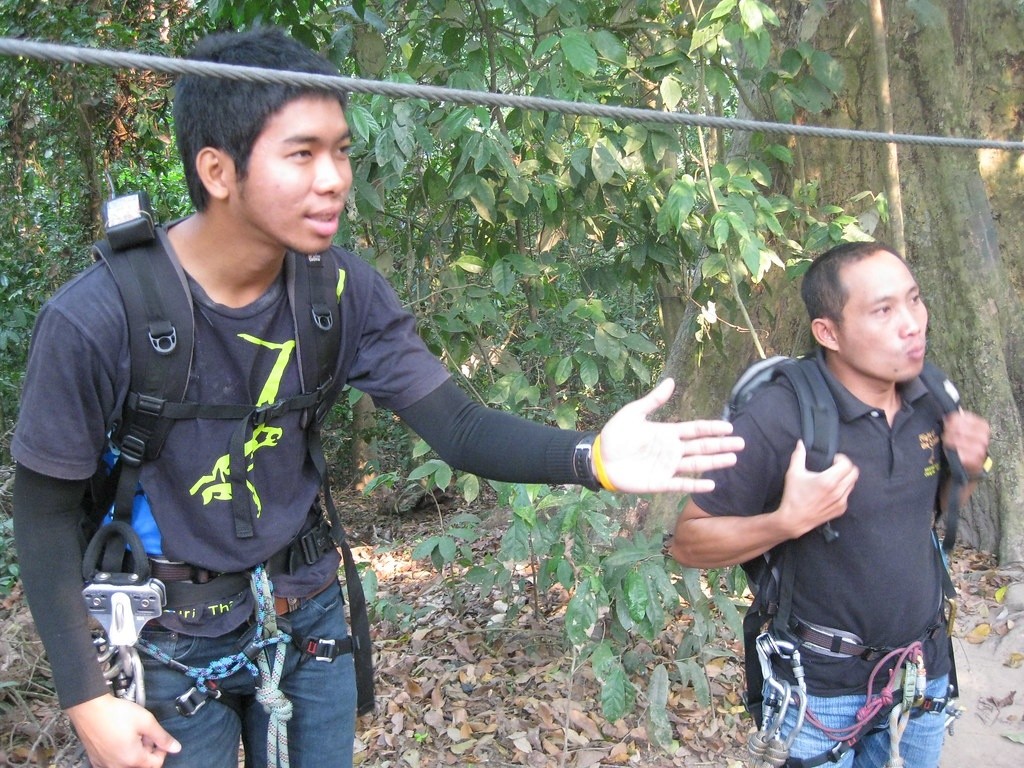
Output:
593;434;618;493
959;452;994;484
574;433;604;492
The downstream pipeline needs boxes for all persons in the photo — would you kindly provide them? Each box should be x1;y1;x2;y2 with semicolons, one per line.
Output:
9;30;748;768
671;240;993;768
